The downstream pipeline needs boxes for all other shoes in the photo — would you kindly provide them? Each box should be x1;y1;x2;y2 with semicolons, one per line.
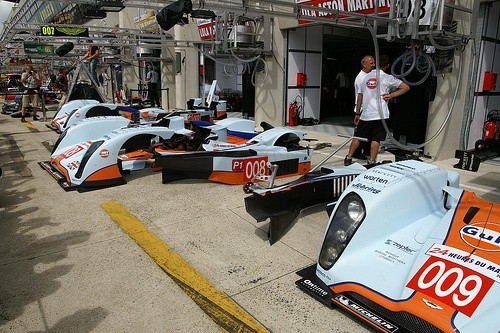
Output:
33;116;36;120
344;154;352;165
21;118;25;122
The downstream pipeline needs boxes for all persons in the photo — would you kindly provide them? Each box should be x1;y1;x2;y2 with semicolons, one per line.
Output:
20;64;41;123
56;61;78;109
98;70;111;96
351;55;376;159
344;55;409;167
145;66;160;108
79;39;101;88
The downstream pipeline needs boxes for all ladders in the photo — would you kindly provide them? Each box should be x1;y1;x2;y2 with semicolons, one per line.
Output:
65;61;110;104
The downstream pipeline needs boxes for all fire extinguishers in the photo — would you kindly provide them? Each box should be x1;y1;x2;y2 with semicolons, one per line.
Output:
289;95;302;126
483;117;496;140
